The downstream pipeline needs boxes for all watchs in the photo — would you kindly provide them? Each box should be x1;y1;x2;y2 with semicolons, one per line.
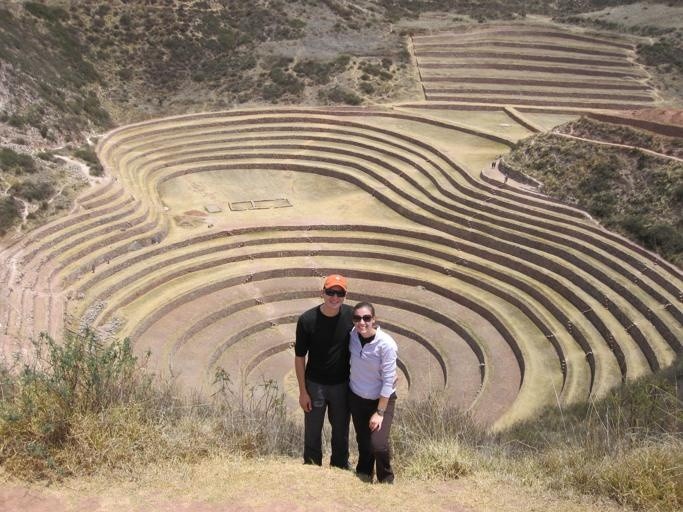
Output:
376;408;386;416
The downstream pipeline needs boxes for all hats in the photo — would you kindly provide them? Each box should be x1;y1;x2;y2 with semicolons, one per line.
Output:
325;274;347;291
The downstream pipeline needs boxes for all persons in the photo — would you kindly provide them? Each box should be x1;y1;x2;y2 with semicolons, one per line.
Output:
346;300;399;486
292;271;399;473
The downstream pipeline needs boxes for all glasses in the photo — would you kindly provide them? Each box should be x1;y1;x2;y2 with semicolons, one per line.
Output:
324;291;347;296
353;314;375;322
